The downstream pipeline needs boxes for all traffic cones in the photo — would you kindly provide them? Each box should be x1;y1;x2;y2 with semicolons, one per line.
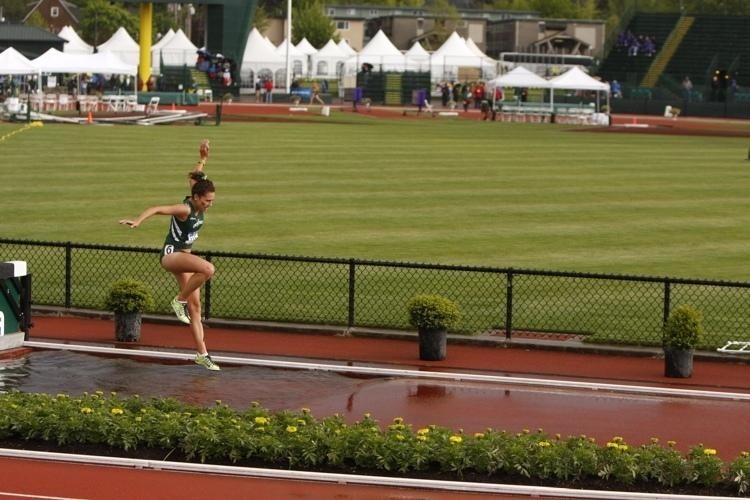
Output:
87;112;94;123
171;101;176;111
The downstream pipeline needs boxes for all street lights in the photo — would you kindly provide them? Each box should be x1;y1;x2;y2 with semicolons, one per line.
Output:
167;4;182;24
187;3;197;40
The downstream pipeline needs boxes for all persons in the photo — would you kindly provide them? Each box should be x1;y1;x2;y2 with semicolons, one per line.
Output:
116;137;221;372
613;28;658;58
264;77;274;103
0;73;158;97
308;80;325;104
254;75;261;102
574;75;623;98
682;74;693;89
492;87;502;101
438;79;486;113
195;46;241;85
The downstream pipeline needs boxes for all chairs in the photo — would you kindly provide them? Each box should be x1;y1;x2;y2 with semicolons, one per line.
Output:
17;91;160;116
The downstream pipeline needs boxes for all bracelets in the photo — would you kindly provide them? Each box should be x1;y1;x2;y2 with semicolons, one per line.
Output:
197;160;207;166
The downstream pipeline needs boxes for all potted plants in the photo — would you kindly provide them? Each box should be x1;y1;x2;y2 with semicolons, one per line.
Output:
102;278;156;343
407;293;461;361
661;304;705;378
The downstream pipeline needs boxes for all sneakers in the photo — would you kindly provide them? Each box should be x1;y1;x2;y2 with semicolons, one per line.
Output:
170;295;192;325
195;352;221;370
